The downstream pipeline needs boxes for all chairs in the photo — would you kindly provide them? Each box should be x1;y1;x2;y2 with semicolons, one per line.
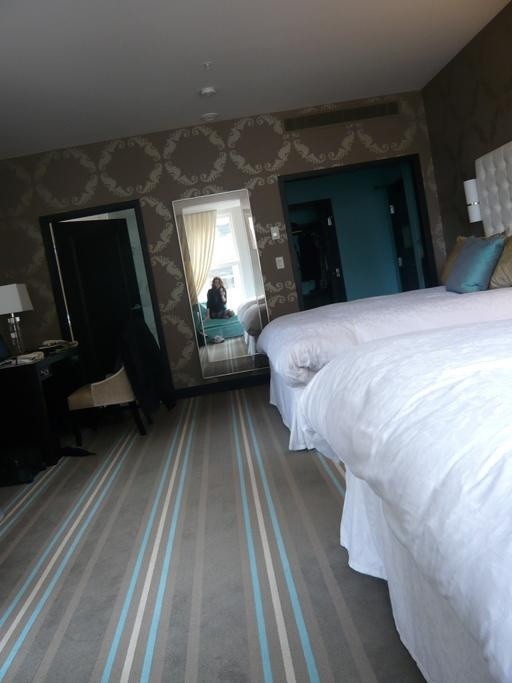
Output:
197;299;245;339
64;311;145;439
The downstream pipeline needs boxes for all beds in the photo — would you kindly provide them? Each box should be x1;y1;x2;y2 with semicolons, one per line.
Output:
236;293;266;345
282;318;510;682
252;276;510;455
242;301;269;354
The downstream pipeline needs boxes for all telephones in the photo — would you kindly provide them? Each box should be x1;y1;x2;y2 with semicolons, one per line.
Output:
43;339;67;347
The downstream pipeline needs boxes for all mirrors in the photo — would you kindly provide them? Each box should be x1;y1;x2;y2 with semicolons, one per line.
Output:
164;183;277;381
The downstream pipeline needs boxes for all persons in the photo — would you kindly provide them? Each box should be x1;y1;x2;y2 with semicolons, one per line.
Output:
205;277;235;319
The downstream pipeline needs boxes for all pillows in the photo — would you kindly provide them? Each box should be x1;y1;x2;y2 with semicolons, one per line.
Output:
435;232;511;290
442;225;510;293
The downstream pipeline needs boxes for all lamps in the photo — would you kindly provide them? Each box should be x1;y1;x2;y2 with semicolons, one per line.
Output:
461;175;480;224
1;279;41;353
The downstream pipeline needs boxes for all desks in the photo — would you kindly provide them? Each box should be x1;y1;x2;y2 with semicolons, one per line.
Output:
1;340;80;489
